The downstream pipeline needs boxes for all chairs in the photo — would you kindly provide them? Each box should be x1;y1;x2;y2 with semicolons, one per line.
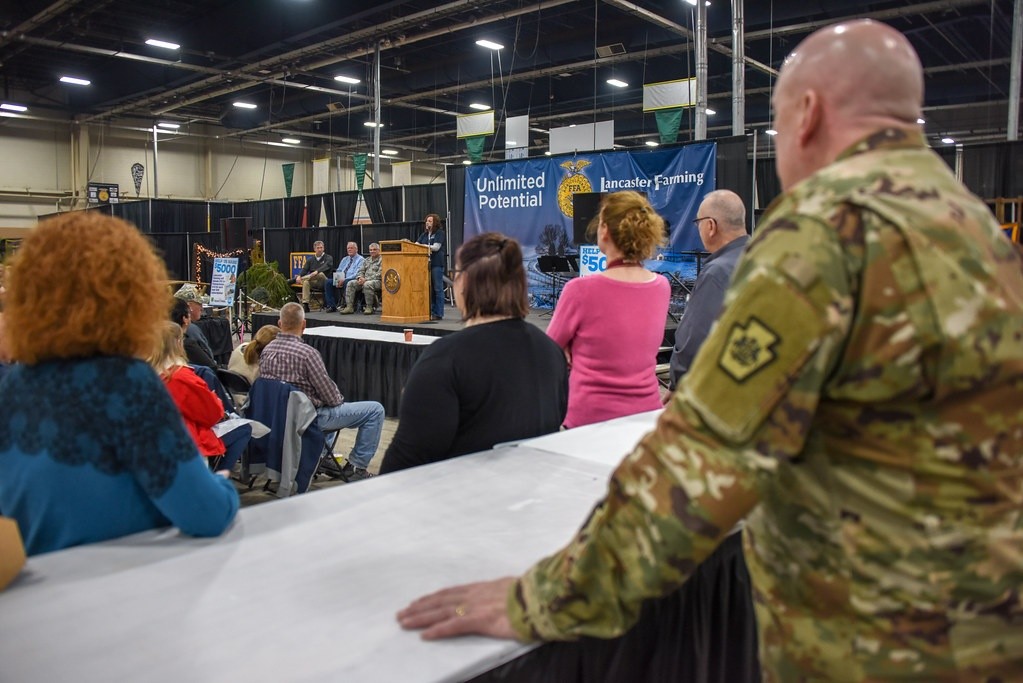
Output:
207;369;348;495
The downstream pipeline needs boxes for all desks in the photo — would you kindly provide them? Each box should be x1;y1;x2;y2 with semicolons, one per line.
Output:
1;410;765;683
303;324;443;419
199;318;233;368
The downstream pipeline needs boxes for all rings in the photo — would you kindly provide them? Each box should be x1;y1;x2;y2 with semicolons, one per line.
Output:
456;606;464;615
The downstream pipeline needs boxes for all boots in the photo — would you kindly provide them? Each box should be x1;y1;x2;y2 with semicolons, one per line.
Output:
363;304;375;315
340;303;354;314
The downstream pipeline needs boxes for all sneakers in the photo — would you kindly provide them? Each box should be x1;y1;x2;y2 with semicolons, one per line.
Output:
316;456;340;473
341;458;377;483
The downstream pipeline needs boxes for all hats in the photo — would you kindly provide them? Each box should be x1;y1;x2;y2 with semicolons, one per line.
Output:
174;284;210;303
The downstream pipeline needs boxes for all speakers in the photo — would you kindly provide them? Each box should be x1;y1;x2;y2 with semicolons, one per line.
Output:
220;217;253;252
573;191;606;244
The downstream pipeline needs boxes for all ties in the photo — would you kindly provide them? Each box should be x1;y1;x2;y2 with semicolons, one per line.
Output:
344;256;353;278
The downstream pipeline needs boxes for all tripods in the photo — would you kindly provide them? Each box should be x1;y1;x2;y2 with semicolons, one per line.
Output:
232;251;252;339
537;255;570;316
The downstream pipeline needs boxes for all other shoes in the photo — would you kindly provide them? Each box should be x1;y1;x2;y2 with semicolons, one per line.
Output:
287;278;295;286
303;303;310;312
429;314;442;320
228;475;249;490
326;307;336;313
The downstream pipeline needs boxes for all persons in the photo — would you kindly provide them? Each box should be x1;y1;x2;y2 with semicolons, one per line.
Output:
287;241;333;312
340;243;382;314
174;284;214;359
228;324;281;384
171;297;217;371
379;232;567;473
324;242;366;313
149;320;252;469
546;190;671;431
259;302;385;481
396;17;1023;683
661;189;751;406
402;214;446;320
1;209;244;556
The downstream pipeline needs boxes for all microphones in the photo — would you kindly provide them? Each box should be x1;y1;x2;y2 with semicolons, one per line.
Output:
428;226;431;236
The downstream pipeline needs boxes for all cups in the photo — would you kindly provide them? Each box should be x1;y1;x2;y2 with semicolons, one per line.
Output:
333;453;342;467
403;329;413;342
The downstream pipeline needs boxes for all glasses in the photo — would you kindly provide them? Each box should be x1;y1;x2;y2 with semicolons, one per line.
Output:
692;217;717;229
